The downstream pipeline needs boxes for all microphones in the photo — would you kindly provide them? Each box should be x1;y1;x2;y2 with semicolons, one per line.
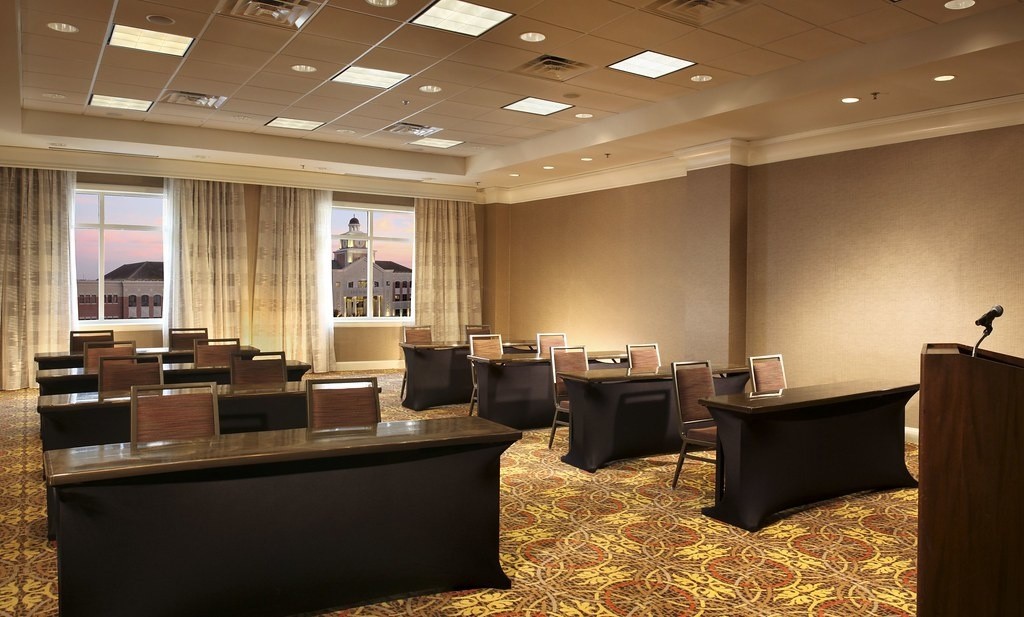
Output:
975;305;1004;326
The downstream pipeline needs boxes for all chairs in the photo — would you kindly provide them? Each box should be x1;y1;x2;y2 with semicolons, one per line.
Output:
131;382;220;443
672;361;718;489
401;326;432;399
83;340;137;367
70;331;114;350
306;377;382;427
627;343;662;368
169;328;208;346
536;332;567;353
468;334;505;416
465;324;491;341
549;346;589;449
98;354;164;391
229;350;288;384
194;338;241;363
747;353;788;392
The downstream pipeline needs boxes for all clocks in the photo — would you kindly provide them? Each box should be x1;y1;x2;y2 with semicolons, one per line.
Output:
353;240;366;248
343;240;348;248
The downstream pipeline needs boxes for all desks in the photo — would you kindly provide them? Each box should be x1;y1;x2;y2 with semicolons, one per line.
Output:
37;381;382;481
34;346;260;370
556;364;750;474
399;340;537;411
42;417;522;617
36;360;311;438
467;351;628;429
699;378;920;533
